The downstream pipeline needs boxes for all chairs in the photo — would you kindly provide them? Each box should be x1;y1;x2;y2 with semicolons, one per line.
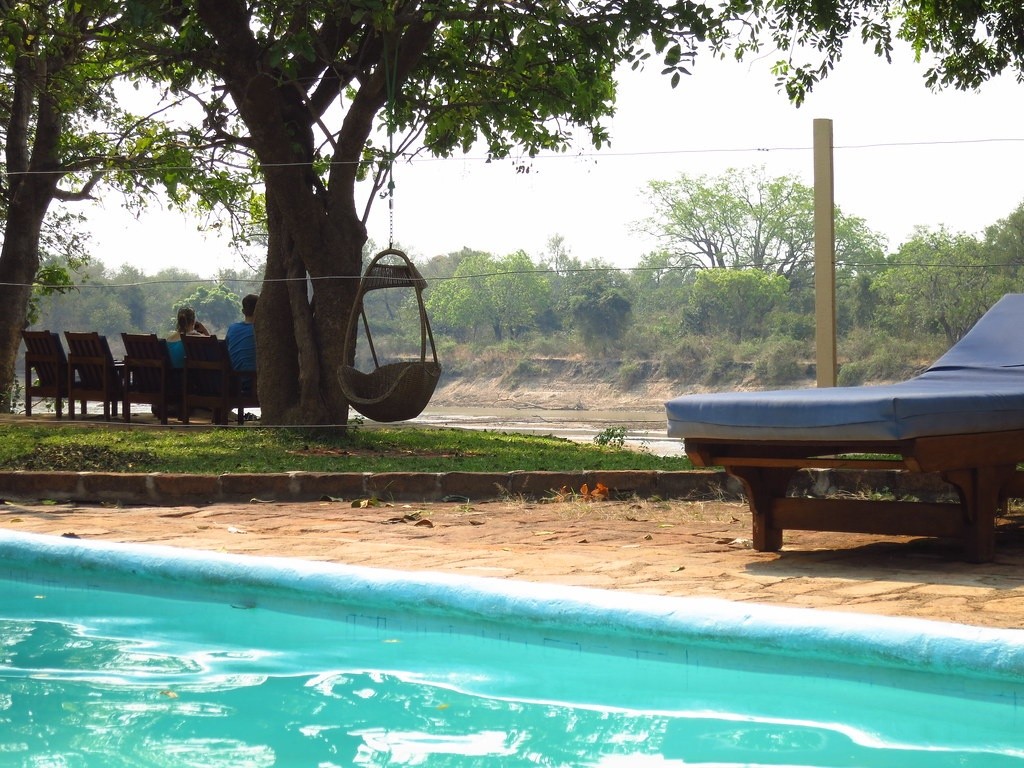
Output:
663;291;1024;564
19;329;260;426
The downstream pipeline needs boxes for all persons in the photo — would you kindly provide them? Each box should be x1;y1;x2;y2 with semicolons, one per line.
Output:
225;294;257;372
166;307;210;341
